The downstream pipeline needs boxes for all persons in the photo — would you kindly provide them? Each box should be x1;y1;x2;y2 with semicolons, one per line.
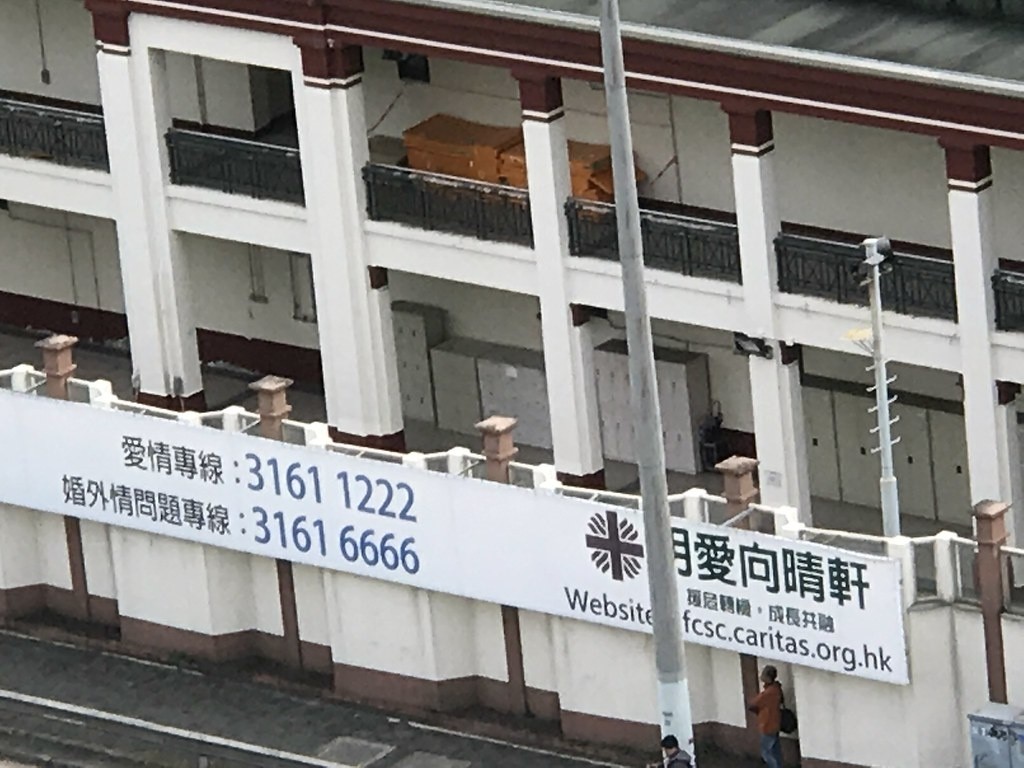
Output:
751;665;786;768
661;735;691;768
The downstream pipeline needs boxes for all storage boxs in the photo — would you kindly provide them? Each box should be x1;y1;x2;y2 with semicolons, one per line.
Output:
399;113;649;221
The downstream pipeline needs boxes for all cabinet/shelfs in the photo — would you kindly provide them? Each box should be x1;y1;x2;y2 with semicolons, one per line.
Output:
389;300;712;478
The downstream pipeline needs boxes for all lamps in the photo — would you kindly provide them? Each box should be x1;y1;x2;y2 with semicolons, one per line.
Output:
732;332;771;359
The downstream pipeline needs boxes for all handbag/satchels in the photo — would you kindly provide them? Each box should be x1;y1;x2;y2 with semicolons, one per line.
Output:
778;708;797;734
